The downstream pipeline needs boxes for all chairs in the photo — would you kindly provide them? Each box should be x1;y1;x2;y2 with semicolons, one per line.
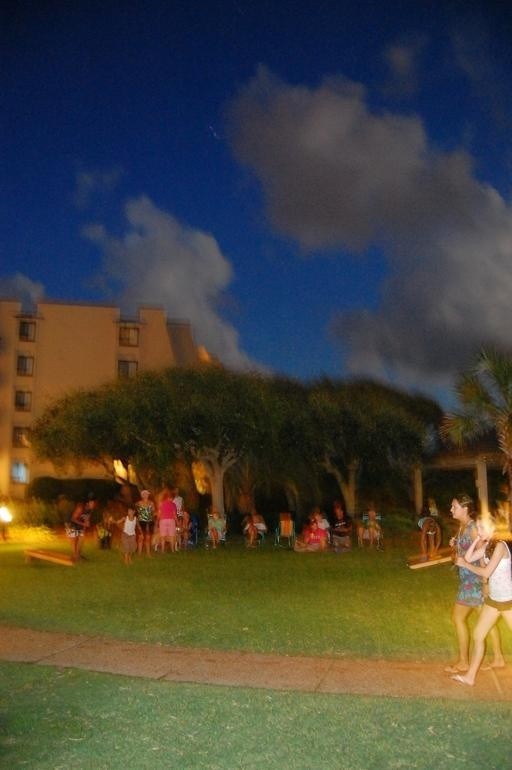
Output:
151;511;383;552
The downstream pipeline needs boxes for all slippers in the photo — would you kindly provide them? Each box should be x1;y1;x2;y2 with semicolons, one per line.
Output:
444;664;468;673
480;663;505;672
452;674;475;687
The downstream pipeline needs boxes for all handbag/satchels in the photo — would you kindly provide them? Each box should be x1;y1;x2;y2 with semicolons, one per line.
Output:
450;531;459;563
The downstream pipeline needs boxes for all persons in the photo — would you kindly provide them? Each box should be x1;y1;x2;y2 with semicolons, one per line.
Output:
444;493;505;674
64;483;383;565
417;516;442;562
450;510;511;686
